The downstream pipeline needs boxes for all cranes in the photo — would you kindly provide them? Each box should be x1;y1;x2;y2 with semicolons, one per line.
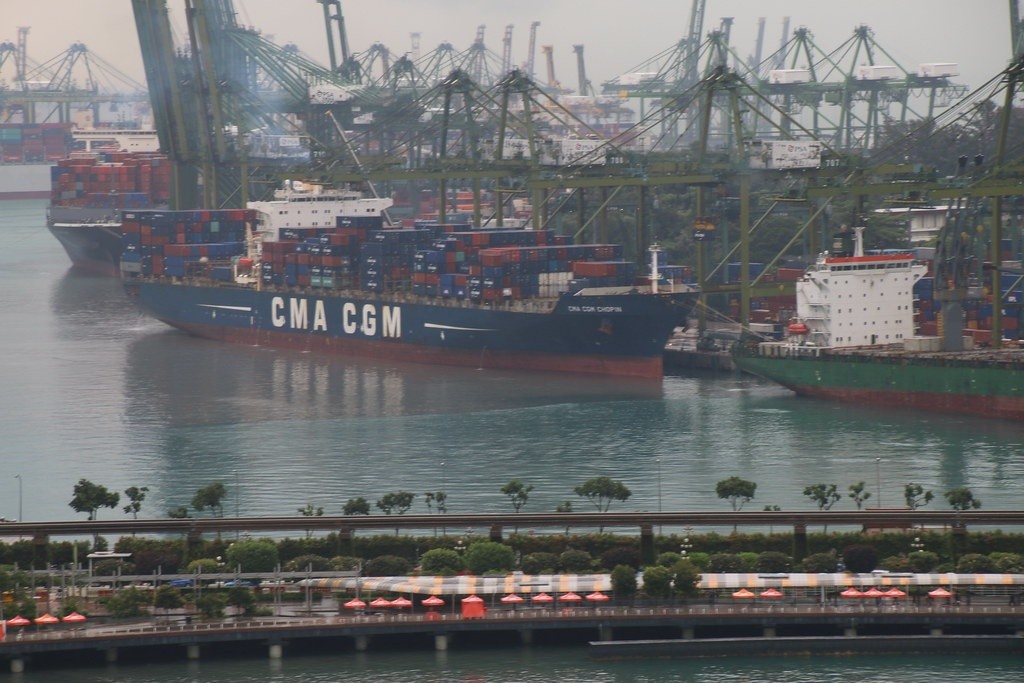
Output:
2;1;1024;285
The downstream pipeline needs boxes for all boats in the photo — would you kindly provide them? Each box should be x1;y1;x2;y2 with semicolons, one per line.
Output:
729;219;1024;423
44;201;168;280
116;174;705;381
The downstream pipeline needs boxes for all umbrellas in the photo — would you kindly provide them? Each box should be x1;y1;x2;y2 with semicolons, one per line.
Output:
35;614;61;631
501;594;523;608
560;592;583;606
586;593;609;605
532;593;554;606
928;587;952;605
369;597;412;613
462;595;482;602
423;596;445;610
7;616;31;632
760;588;783;603
841;587;905;606
63;613;87;628
733;589;754;602
344;599;366;615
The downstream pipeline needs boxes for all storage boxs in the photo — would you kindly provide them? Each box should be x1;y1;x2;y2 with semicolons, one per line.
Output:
918;63;959;77
713;262;805;323
120;210;257;284
0;121;170;207
859;65;898;80
502;123;637;166
912;238;1024;348
259;216;696;306
